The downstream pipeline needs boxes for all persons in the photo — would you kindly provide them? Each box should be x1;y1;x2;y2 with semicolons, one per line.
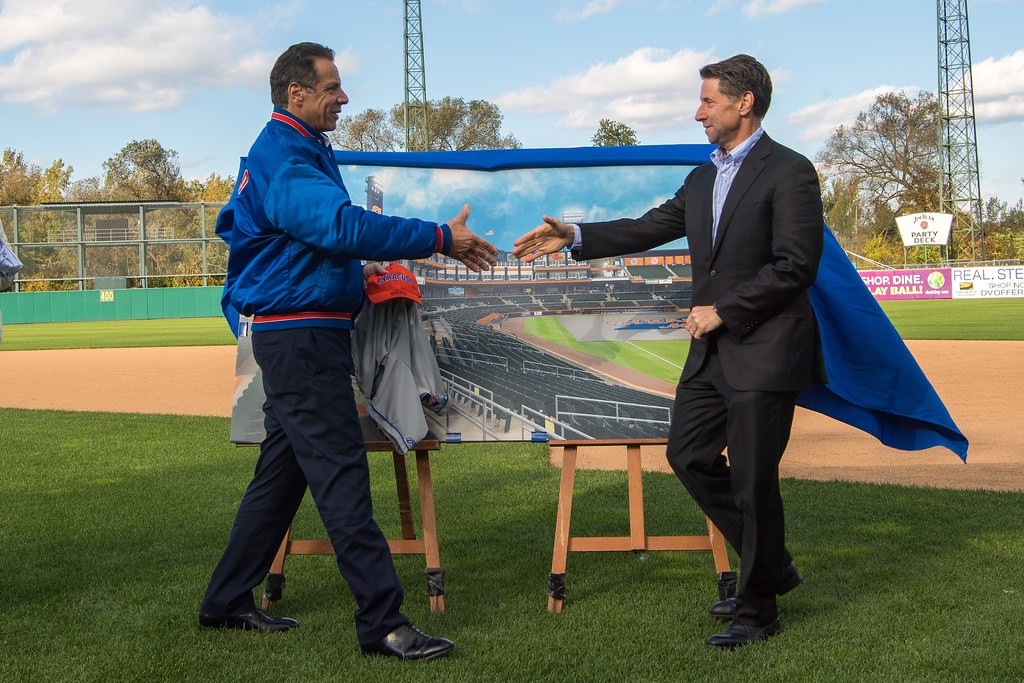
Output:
511;53;819;645
196;41;496;661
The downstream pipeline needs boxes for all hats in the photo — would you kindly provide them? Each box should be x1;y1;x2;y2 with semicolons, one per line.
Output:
364;263;423;306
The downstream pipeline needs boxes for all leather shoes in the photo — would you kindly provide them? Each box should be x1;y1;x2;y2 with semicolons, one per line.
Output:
708;566;802;616
200;608;298;633
361;624;456;661
707;616;780;651
